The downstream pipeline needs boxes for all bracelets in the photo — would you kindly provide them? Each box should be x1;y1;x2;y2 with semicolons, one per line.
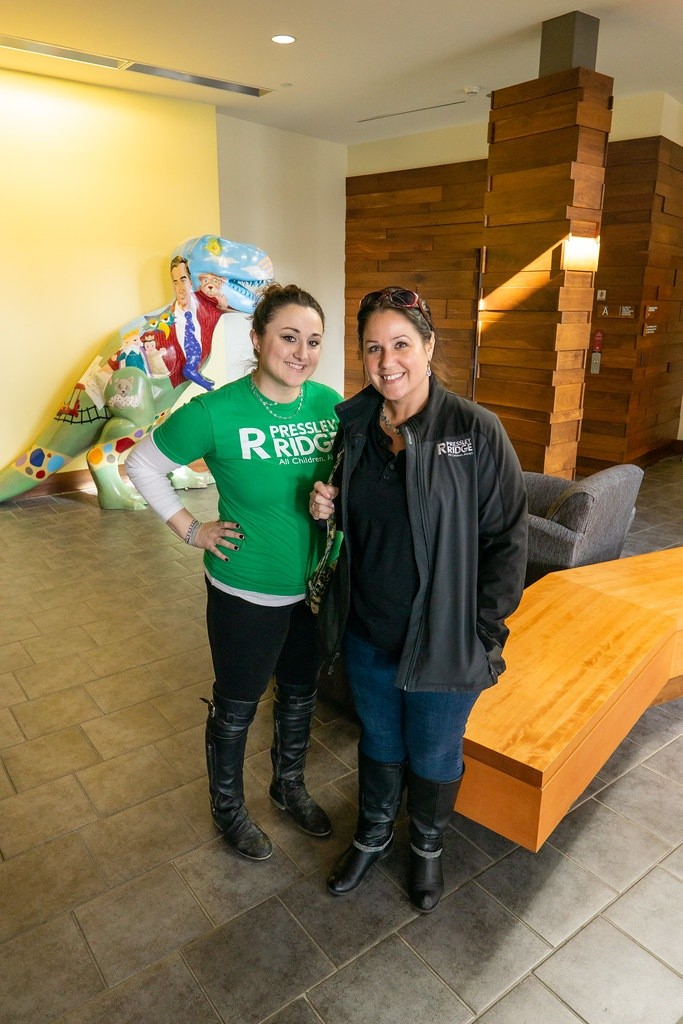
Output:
184;517;203;546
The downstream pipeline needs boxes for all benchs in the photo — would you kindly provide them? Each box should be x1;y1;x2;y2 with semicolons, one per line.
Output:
454;546;683;855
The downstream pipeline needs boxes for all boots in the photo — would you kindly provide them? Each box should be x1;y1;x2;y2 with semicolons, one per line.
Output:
327;743;408;895
197;681;274;861
268;684;333;836
404;759;467;913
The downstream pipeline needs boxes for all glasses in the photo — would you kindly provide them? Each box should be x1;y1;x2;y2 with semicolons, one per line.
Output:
358;289;432;330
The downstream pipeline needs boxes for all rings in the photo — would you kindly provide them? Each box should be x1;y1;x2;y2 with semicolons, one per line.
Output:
310;501;317;510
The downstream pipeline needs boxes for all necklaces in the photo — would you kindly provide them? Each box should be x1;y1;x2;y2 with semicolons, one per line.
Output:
249;367;304;421
379;396;404;437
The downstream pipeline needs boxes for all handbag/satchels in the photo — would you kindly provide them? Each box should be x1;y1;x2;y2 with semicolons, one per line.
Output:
304;557;339;616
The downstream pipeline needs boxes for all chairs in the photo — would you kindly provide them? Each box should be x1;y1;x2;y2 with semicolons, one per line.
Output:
522;463;645;589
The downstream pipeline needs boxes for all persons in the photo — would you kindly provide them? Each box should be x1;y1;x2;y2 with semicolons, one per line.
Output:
124;278;349;863
308;284;533;918
